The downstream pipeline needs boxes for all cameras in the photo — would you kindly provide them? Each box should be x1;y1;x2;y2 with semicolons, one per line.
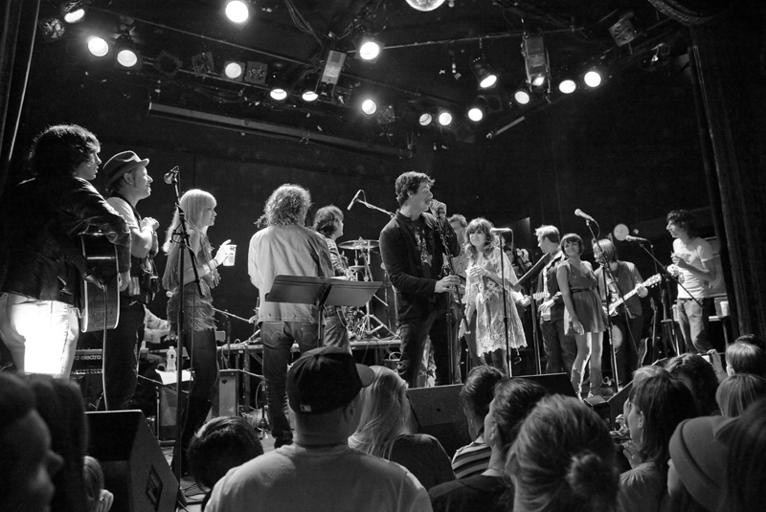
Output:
701;353;713;365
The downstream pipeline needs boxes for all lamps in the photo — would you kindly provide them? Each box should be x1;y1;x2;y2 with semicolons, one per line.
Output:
269;70;287;100
558;70;577;93
112;35;140;69
468;97;486;122
584;66;606;87
436;106;451;125
471;56;497;89
63;1;85;22
515;86;533;105
223;2;250;27
412;102;432;126
80;35;107;63
222;61;245;82
300;77;318;101
356;30;382;61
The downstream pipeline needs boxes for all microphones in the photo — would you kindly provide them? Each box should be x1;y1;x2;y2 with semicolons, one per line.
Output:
346;188;363;210
625;233;649;245
489;226;513;235
162;164;179;185
574;208;596;225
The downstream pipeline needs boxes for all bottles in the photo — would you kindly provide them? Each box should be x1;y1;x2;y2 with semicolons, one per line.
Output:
166;345;176;373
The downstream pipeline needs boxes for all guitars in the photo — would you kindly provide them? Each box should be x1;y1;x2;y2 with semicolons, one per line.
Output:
601;273;662;318
77;229;122;333
637;297;663;367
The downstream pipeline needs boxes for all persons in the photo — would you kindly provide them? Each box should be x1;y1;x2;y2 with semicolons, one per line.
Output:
379;170;460;388
0;121;134;380
0;329;765;510
447;207;719;403
245;181;337;449
90;148;162;412
162;187;235;477
310;202;353;358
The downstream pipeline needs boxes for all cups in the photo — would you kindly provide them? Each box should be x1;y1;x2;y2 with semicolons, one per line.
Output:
463;267;480;286
218;244;238;266
719;300;730;317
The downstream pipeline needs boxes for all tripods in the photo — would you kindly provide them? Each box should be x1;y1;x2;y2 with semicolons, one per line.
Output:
350;258;400;340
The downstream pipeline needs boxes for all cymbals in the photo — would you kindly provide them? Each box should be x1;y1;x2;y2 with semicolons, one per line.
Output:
338;239;381;250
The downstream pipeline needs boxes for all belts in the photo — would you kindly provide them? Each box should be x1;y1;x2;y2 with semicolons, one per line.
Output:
571;287;593;293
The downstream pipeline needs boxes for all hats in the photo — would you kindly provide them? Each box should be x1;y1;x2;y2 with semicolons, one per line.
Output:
285;348;378;416
667;413;731;509
102;150;150;191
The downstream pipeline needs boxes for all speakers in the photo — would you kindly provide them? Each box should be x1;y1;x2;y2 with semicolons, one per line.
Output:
607;356;668;430
511;372;578;399
84;408;181;512
406;384;473;452
